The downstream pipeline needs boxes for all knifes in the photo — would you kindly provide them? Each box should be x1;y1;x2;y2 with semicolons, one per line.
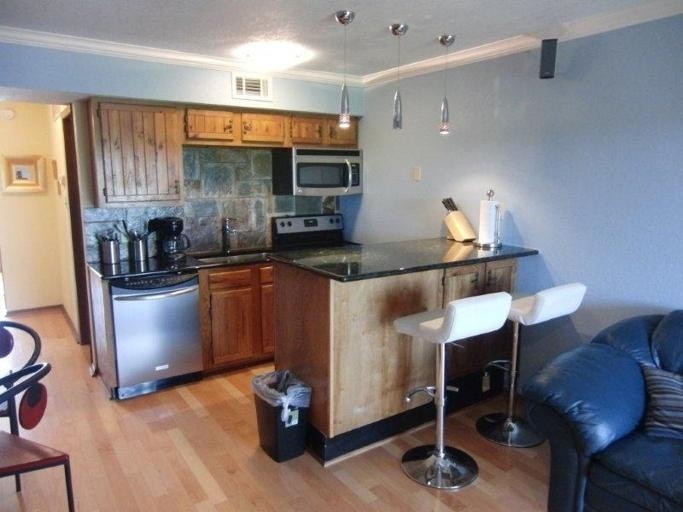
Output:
442;197;457;212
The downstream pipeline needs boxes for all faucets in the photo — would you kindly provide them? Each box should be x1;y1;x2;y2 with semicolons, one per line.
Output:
222;217;237;251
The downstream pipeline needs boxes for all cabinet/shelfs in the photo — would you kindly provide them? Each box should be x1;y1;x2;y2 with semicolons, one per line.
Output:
182;106;287;150
286;113;359;149
199;260;273;375
87;98;185;208
444;258;523;417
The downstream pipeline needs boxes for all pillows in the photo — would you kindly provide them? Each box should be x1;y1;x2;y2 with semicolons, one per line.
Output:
643;362;682;440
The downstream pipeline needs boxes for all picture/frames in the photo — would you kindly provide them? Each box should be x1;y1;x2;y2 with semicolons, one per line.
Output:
1;155;45;194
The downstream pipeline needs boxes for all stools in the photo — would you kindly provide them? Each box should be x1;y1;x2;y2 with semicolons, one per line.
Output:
393;291;512;491
476;281;587;448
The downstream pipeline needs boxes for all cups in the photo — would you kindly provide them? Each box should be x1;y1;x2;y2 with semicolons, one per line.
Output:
101;240;120;264
131;239;148;261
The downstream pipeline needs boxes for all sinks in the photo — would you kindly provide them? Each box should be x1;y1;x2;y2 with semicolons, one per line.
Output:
197;253;272;265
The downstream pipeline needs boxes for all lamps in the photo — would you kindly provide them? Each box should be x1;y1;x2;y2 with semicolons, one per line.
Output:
334;10;357;128
388;24;407;132
435;34;456;135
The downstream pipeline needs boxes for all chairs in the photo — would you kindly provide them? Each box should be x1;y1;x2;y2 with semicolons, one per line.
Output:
0;363;75;512
0;321;42;491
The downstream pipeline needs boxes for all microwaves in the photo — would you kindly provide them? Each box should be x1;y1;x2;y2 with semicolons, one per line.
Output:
273;148;363;196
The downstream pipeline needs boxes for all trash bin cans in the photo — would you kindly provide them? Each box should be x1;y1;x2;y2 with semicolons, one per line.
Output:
251;369;313;463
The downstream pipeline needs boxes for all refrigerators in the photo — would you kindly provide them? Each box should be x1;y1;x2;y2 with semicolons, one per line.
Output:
86;256;204;401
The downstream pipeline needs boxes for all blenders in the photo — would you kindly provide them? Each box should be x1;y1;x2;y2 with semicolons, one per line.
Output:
149;216;191;260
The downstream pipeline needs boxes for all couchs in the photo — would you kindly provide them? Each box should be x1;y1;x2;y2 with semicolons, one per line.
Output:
522;310;683;512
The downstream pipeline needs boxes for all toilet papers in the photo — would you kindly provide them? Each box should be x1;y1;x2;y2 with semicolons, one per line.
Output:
477;200;498;243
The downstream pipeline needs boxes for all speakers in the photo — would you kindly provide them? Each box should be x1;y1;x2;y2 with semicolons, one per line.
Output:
540;39;557;79
